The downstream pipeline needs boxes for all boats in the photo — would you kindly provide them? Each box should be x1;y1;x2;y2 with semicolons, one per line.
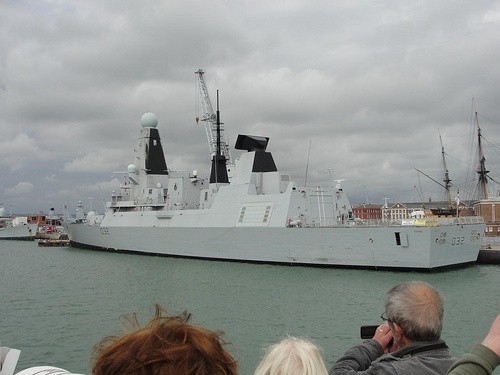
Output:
0;67;500;274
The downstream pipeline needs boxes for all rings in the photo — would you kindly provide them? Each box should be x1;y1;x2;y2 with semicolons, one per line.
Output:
380;329;383;332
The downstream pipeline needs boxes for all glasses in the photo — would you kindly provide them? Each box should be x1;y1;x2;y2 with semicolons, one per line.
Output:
381;312;391;322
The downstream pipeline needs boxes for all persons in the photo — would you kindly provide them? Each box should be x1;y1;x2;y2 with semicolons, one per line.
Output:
446;312;500;375
329;281;457;375
90;304;239;375
251;338;329;375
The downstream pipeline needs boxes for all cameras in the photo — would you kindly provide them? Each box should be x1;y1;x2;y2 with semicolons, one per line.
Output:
361;325;394;347
